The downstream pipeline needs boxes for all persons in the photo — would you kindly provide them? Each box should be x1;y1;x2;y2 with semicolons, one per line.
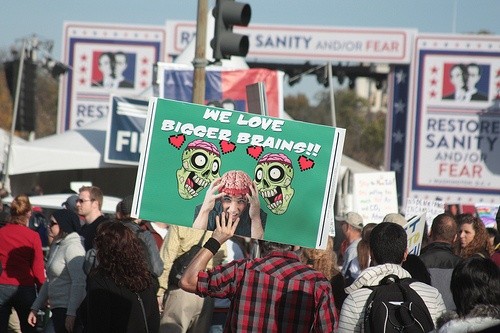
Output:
0;184;164;333
91;51;134;88
441;64;488;102
191;170;267;239
140;219;344;333
336;206;500;333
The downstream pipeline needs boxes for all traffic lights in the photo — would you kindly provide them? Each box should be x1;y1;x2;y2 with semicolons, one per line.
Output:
209;0;252;60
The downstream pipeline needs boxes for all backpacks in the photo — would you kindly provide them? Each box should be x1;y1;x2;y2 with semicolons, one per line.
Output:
363;274;435;333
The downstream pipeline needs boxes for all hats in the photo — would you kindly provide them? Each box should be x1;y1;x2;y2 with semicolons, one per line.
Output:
334;212;363;230
220;170;252;195
383;213;407;228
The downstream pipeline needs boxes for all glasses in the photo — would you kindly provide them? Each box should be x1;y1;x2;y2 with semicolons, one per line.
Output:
50;220;58;227
75;199;94;204
